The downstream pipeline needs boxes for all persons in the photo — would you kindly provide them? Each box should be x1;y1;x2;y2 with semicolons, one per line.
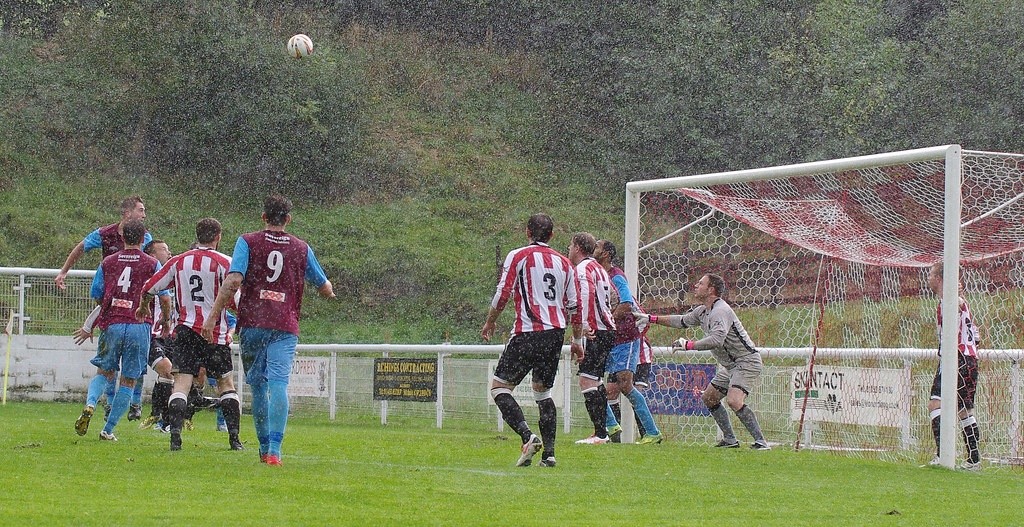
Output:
55;195;153;290
563;231;620;444
920;263;983;470
480;213;585;468
74;219;172;442
591;239;663;445
201;195;336;468
135;217;242;453
630;274;771;451
72;240;237;435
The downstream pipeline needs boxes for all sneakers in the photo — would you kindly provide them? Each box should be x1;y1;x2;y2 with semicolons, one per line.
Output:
182;420;195;431
574;431;610;444
260;453;284;468
170;428;183;452
516;433;543;467
104;404;111;422
191;396;222;413
608;423;622;439
139;414;171;434
919;456;940;467
128;403;142;422
749;441;771;450
635;432;664;445
99;430;117;441
714;438;739;448
75;404;95;436
229;432;247;451
537;456;555;467
957;461;983;471
217;424;228;431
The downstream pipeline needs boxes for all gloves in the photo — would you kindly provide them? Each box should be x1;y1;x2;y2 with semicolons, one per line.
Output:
632;312;650;326
672;337;688;353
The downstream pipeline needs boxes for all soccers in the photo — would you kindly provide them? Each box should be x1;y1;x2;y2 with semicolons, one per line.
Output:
287;34;313;60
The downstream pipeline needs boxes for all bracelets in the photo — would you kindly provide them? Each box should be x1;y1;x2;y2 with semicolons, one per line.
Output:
162;316;170;321
